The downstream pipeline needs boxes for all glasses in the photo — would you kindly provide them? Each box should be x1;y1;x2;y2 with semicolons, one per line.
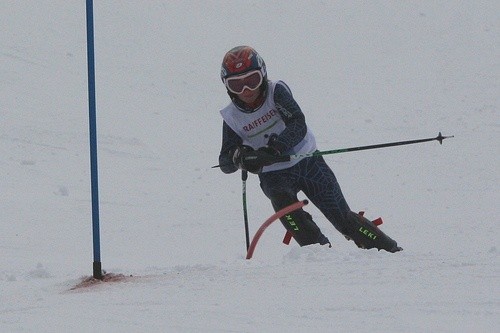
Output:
225;69;264;92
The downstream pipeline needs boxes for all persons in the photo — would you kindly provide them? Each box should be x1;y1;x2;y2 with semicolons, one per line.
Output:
217;44;405;255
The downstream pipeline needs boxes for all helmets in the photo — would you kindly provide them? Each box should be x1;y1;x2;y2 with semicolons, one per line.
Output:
221;45;265;81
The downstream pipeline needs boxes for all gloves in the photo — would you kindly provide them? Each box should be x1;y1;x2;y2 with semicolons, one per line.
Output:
244;145;281;174
233;145;263;173
